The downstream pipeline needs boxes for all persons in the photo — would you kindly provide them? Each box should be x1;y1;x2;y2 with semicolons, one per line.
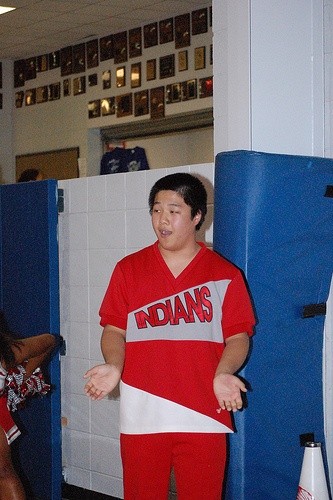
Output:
0;318;57;500
85;172;255;500
